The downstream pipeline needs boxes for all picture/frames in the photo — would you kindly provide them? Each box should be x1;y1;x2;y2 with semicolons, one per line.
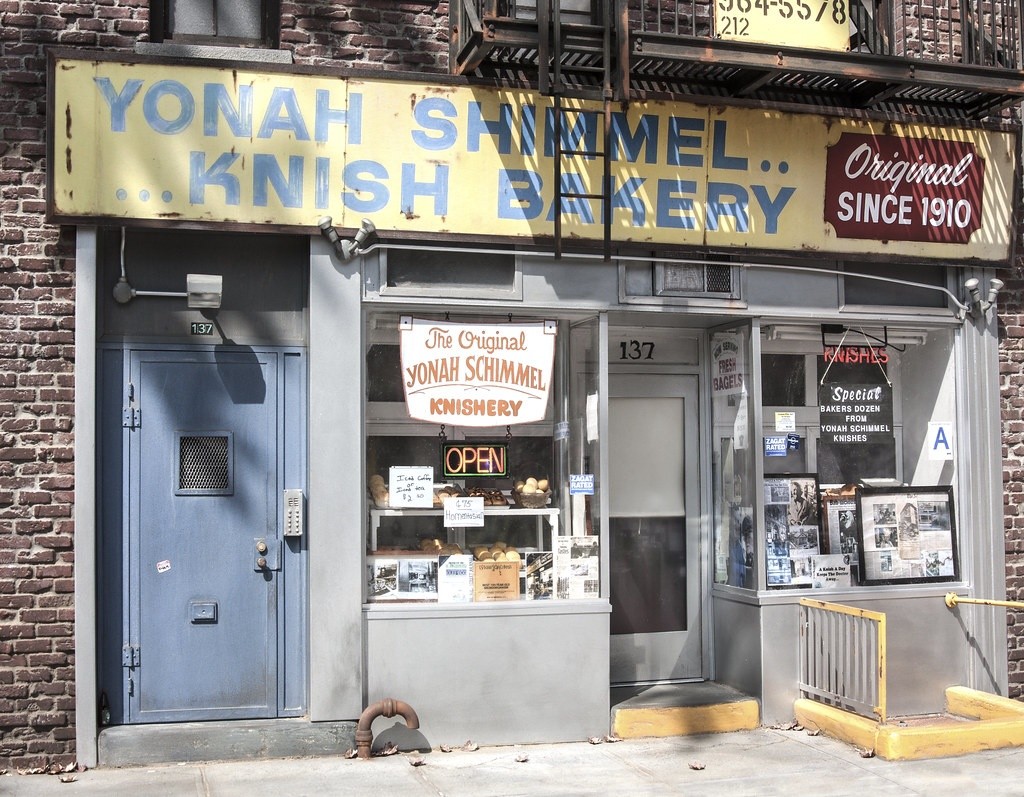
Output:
764;472;961;590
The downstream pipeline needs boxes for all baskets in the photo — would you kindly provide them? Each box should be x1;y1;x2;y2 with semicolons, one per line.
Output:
511;461;553;508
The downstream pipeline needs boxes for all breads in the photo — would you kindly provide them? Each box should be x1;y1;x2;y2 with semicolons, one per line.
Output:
433;485;506;505
420;539;463;556
472;542;520;563
368;475;389;508
514;477;549;495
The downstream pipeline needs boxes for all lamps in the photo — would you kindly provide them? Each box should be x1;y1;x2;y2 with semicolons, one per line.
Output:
318;216;376;261
185;273;223;309
964;277;1004;320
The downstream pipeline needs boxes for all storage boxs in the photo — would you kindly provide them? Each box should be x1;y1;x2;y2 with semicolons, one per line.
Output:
366;548;521;601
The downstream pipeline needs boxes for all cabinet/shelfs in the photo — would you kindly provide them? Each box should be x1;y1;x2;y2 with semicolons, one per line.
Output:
368;505;560;601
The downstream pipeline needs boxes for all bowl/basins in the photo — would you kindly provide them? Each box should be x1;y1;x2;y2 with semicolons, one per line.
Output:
512;490;552;507
